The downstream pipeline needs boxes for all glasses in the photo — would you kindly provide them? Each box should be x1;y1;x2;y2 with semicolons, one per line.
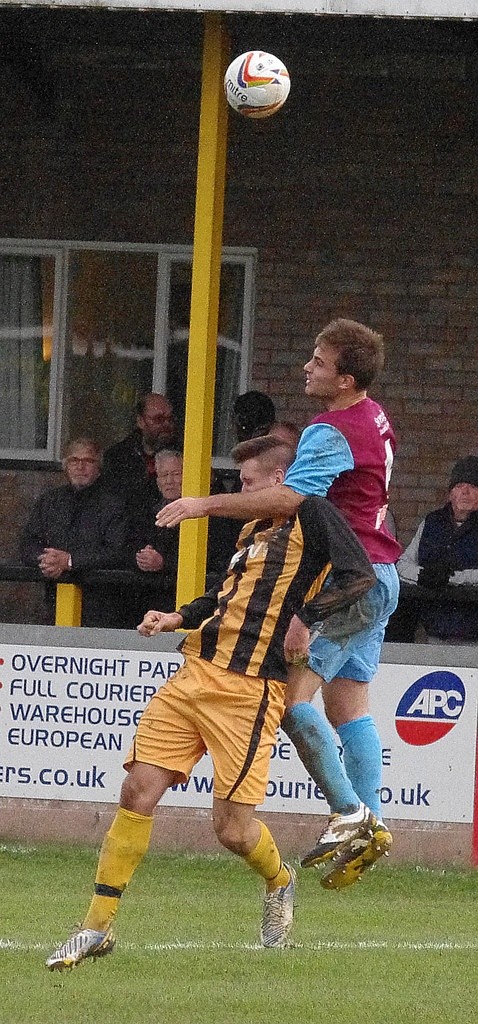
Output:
142;414;176;424
66;456;101;467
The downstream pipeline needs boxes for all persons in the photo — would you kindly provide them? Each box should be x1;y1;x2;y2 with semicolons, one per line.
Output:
17;430;146;629
46;434;378;972
155;316;401;892
223;390;302;545
127;449;237;614
395;456;478;646
98;392;226;502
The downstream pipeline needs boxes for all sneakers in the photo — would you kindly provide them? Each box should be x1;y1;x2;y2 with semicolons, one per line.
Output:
319;821;393;891
45;926;116;972
261;859;297;947
300;801;376;868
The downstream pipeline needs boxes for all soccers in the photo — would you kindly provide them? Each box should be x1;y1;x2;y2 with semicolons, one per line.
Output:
223;48;294;120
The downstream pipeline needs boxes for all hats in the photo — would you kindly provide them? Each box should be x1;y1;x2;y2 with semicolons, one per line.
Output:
234;390;275;442
447;455;478;492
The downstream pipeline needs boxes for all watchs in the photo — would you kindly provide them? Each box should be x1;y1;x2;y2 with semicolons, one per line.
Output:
67;554;72;571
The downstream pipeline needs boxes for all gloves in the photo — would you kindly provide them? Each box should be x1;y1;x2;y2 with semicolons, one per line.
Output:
417;566;456;587
